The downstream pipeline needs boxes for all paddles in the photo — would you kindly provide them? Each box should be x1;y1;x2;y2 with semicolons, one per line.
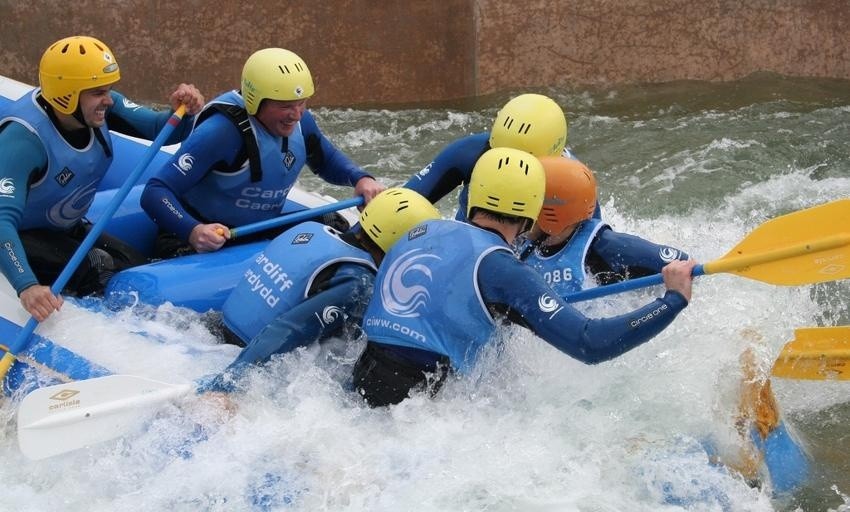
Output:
743;326;850;381
558;197;850;304
16;374;221;461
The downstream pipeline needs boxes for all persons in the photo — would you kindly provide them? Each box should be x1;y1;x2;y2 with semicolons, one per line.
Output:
401;93;600;232
138;47;389;259
194;186;442;421
515;154;697;298
0;35;204;322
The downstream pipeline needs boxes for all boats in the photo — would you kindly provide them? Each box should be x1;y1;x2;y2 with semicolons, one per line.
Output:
0;76;808;510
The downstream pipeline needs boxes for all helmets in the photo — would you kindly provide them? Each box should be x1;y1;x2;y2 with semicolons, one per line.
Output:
240;48;315;116
536;155;597;236
38;36;120;115
359;187;441;254
489;93;567;157
467;147;546;231
